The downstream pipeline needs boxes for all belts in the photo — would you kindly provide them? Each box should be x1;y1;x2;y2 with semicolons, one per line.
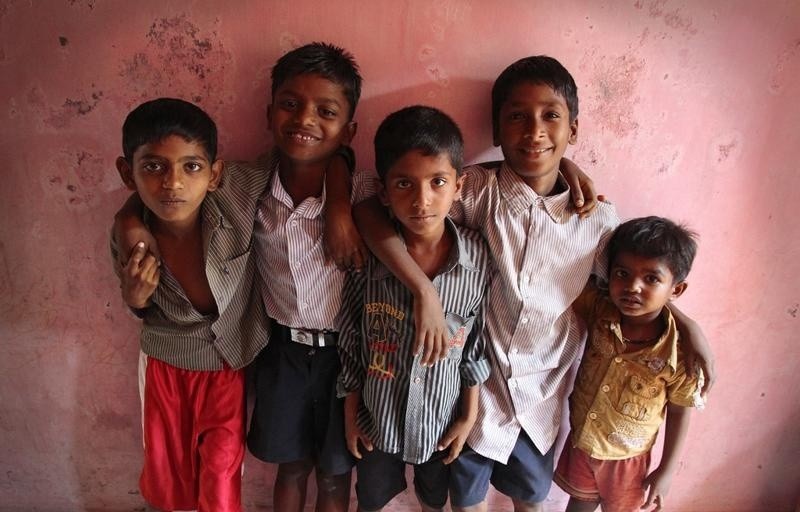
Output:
281;327;337;349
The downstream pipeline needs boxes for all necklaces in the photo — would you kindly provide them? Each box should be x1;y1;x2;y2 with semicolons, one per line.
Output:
621;322;667;345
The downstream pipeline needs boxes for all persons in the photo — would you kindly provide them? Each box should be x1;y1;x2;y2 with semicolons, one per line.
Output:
105;38;604;512
105;94;370;512
349;48;719;512
545;213;703;512
331;102;493;512
